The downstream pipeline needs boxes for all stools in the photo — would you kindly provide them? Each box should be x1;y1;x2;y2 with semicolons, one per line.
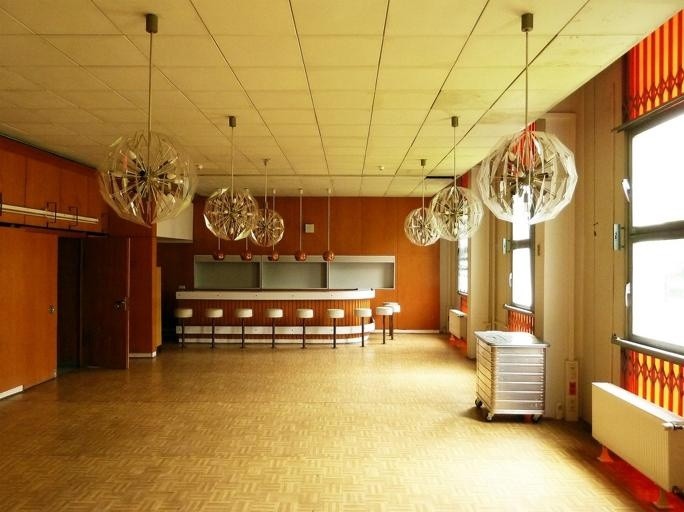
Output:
174;301;401;350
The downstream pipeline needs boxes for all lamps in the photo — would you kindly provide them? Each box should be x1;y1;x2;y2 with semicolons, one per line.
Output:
98;130;197;224
240;250;252;261
404;208;442;247
429;185;484;242
212;249;225;260
323;251;334;261
204;186;258;240
248;208;284;249
268;251;279;261
296;251;306;260
476;131;577;228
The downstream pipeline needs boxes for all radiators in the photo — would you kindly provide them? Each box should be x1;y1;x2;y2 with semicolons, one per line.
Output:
591;381;684;510
449;309;467;340
